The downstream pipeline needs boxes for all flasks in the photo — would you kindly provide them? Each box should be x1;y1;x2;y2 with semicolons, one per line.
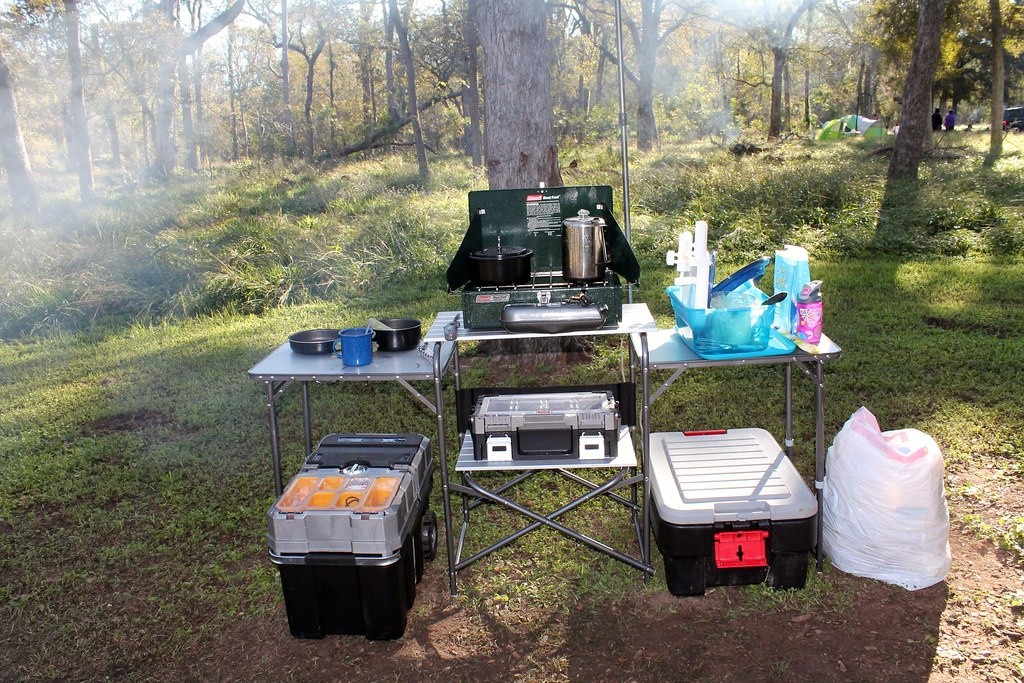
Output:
797;278;825;344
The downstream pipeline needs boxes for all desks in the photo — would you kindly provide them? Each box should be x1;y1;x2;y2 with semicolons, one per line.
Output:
627;327;842;585
246;341;470;597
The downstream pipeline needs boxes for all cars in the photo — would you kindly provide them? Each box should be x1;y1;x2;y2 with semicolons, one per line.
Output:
1003;107;1024;134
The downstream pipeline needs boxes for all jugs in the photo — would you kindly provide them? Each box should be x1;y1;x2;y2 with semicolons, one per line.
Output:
709;287;765;345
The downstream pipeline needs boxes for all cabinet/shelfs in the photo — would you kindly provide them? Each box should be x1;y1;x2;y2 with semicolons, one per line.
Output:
423;302;659;599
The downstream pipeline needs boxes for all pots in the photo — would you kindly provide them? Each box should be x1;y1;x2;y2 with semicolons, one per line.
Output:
470;235;535;285
560;208;612;282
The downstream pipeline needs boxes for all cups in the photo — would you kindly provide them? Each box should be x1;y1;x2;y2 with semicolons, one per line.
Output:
333;326;374;366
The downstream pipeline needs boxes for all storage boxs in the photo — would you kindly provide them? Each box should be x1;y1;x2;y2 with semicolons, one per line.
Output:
640;427;817;598
469;390;622;462
665;282;777;355
264;432;439;642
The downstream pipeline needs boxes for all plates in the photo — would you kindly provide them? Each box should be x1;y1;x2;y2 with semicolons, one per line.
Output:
707;255;773;308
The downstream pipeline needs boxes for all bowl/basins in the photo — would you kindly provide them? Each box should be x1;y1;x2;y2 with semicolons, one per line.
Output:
371;317;422;351
288;329;345;353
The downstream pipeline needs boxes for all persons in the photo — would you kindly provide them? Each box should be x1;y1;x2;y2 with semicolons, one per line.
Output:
932;108;942;131
944;110;956;131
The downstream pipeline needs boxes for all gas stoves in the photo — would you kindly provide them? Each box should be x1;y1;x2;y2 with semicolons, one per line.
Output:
455;255;623;330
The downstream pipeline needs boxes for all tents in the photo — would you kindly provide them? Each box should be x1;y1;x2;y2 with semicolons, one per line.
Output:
817;113;889;142
